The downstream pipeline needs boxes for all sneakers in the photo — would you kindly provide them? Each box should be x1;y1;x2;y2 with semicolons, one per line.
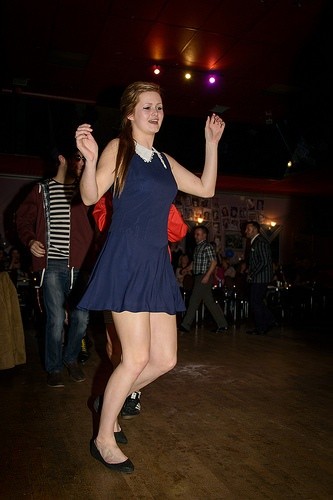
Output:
67;365;86;382
45;372;64;387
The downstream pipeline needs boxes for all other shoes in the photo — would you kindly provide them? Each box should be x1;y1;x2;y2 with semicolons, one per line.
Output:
120;393;140;419
216;327;230;334
177;325;190;333
246;329;266;335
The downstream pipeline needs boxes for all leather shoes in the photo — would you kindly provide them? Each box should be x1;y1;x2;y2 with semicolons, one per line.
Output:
93;395;128;444
90;438;135;474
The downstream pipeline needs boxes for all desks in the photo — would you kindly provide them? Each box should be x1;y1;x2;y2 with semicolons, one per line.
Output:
278;285;333;325
0;268;28;371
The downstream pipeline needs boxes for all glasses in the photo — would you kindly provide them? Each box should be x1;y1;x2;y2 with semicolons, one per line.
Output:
72;155;86;161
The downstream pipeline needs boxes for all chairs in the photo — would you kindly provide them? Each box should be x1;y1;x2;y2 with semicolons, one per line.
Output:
180;274;250;327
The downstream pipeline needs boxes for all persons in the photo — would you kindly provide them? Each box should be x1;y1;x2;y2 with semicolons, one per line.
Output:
211;264;224;311
6;246;22;274
8;137;92;387
177;254;190;302
74;81;225;474
176;225;229;335
245;221;273;335
88;186;188;418
